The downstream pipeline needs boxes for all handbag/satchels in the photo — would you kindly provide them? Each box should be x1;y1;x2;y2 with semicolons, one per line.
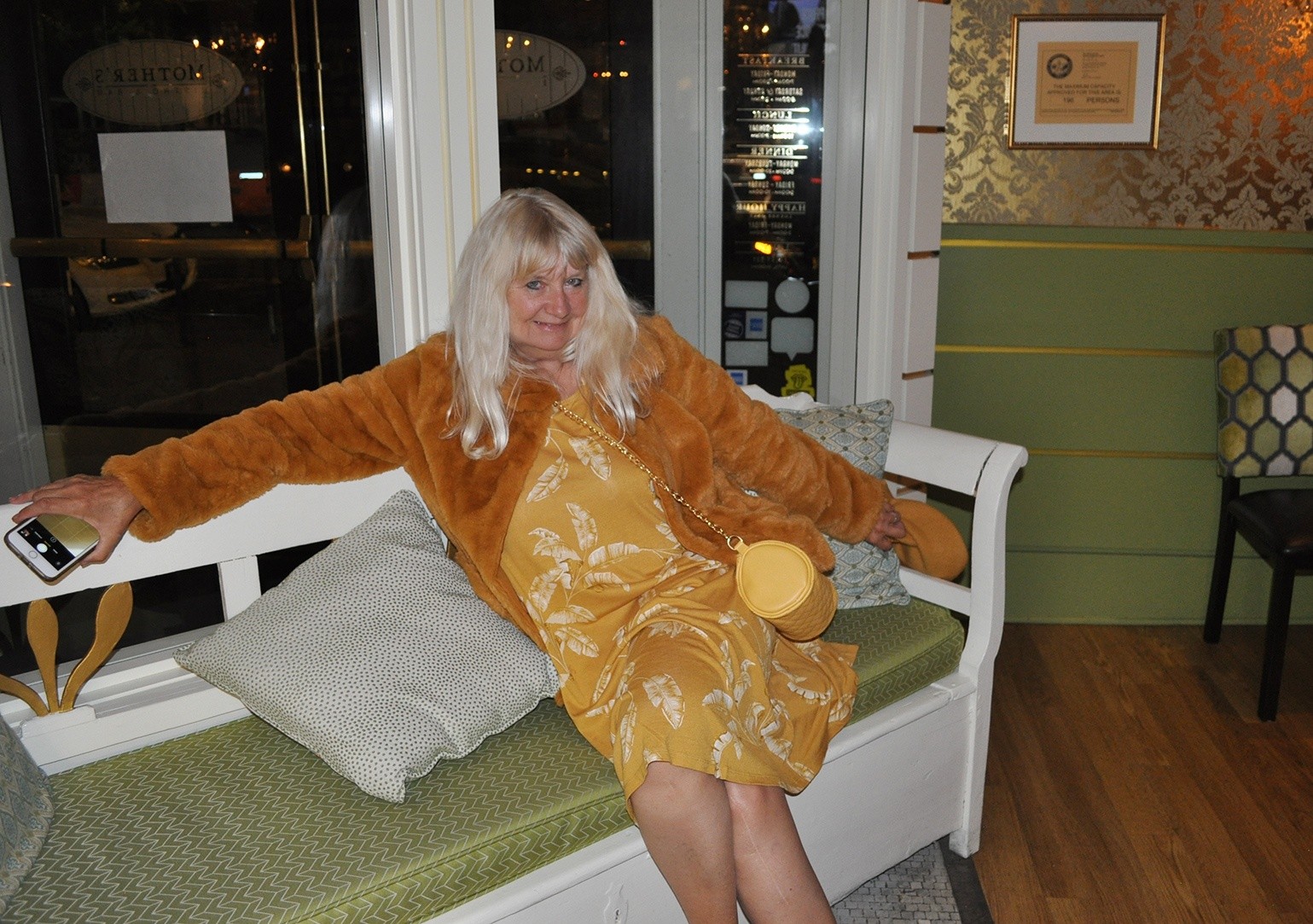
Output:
735;541;838;641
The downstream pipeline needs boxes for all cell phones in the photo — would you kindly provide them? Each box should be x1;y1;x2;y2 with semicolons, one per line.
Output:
4;512;100;581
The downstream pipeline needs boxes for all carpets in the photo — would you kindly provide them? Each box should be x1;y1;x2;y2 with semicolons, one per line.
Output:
829;837;995;924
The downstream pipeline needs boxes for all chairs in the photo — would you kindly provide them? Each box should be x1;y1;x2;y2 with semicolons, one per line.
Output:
1202;321;1313;722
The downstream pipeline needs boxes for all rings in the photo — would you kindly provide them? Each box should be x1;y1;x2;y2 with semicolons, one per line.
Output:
891;511;897;526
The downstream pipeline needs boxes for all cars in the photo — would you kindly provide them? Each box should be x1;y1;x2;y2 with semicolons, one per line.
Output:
59;202;199;326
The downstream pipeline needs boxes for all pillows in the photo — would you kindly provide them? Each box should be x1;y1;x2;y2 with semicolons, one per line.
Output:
747;398;912;610
172;489;559;804
0;716;54;914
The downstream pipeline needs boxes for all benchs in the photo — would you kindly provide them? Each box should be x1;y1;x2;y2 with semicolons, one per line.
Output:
0;383;1028;924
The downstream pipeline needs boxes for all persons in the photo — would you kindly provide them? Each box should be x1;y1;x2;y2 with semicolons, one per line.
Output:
8;190;907;924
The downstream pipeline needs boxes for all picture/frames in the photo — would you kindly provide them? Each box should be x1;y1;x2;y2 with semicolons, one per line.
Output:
1008;14;1166;151
